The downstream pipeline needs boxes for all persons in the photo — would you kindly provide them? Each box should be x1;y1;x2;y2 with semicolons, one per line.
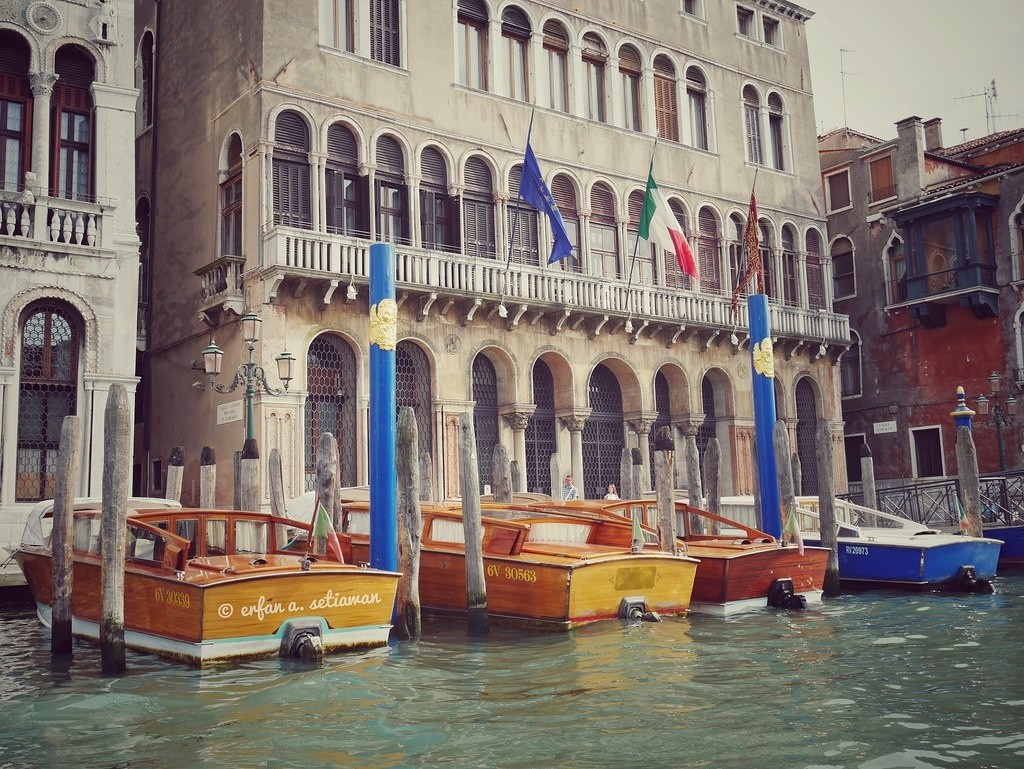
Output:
562;476;579;501
605;485;619;499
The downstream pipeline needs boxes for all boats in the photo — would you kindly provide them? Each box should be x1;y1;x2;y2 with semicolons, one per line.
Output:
676;496;1005;597
286;485;701;635
1;495;404;670
527;500;834;619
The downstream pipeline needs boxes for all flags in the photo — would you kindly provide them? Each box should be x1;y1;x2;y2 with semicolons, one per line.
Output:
785;508;804;556
956;498;970;529
637;159;699;281
311;503;344;564
519;140;573;265
634;515;646;553
733;191;764;321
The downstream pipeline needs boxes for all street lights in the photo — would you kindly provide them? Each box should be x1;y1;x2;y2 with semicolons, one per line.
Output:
202;308;298;458
973;371;1018;511
